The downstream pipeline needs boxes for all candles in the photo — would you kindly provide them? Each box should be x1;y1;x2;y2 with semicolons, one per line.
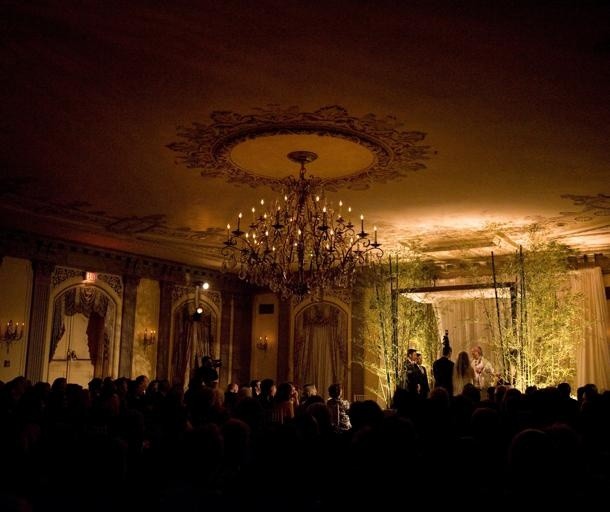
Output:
6;321;25;337
144;329;156;340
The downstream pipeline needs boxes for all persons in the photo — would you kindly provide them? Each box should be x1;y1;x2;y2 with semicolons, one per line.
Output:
197;356;218;382
1;375;610;512
403;344;495;401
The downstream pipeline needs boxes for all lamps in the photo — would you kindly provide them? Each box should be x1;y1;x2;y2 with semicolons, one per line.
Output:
220;151;383;307
